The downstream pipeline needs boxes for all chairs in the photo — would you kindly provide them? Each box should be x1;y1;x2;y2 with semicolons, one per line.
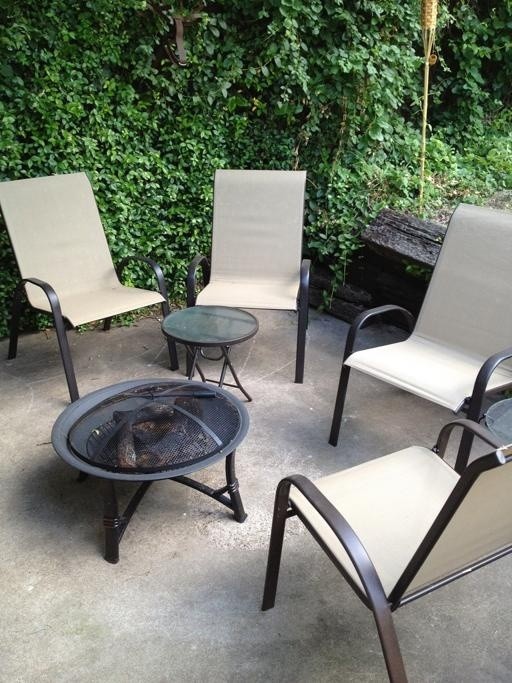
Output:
262;419;511;683
329;204;510;471
0;169;179;403
185;168;313;383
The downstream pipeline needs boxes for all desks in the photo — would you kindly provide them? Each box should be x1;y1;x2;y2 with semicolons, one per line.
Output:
485;399;510;446
163;306;259;403
